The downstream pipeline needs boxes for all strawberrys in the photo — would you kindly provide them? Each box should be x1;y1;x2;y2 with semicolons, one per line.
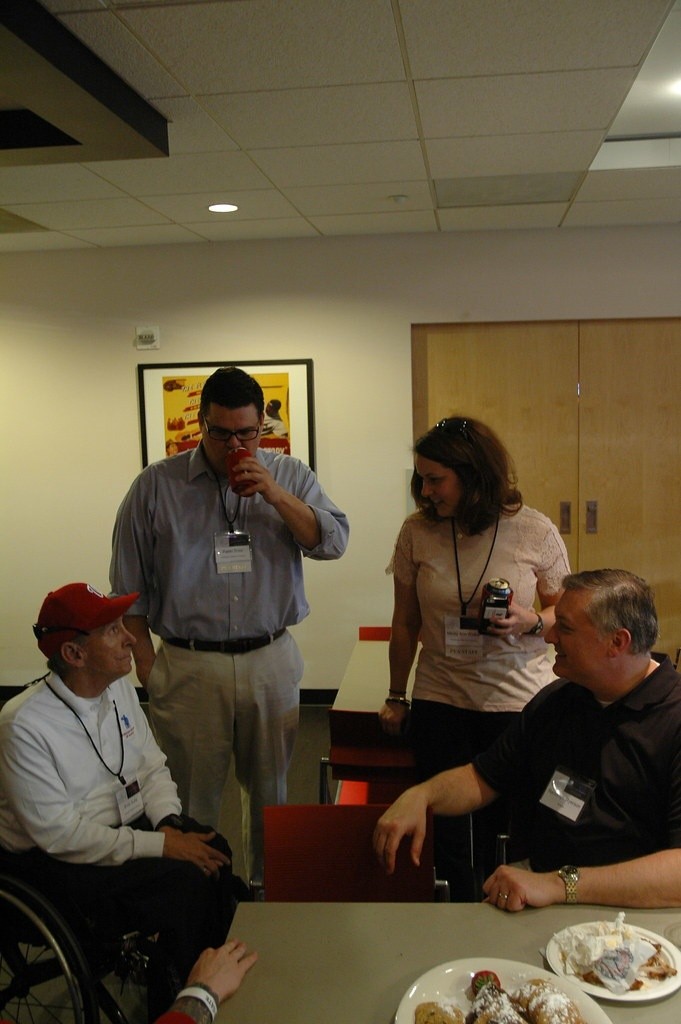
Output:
472;971;501;996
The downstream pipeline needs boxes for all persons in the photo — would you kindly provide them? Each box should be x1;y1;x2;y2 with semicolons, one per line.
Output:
372;568;681;912
109;367;350;886
0;582;252;1024
378;415;572;903
154;938;258;1024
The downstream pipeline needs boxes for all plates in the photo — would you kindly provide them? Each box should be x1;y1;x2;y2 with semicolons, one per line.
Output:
545;923;681;1000
394;958;614;1024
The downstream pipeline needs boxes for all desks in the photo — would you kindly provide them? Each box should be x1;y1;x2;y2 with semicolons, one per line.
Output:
331;640;423;711
212;903;681;1024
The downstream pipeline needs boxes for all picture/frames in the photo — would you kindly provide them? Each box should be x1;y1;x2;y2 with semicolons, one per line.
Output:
137;357;315;471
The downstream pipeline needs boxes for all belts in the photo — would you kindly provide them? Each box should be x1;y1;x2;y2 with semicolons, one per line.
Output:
158;626;290;654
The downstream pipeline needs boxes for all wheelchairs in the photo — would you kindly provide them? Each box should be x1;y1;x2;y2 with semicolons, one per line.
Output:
1;870;163;1024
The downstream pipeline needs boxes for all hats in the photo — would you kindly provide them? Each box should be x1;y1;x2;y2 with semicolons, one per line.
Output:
36;581;142;658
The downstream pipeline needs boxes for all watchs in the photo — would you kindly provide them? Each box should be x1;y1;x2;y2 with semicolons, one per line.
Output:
559;865;580;903
529;614;543;635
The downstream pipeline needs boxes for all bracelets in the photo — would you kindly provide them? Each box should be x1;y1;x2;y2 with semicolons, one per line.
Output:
175;987;217;1024
384;689;410;707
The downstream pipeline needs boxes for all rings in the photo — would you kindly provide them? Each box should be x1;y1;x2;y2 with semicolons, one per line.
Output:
498;892;507;899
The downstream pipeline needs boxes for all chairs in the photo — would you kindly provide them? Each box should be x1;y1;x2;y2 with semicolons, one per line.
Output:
359;626;392;641
263;709;450;903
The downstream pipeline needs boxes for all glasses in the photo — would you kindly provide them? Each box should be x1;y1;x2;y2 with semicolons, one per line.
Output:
200;413;263;441
31;622;89;639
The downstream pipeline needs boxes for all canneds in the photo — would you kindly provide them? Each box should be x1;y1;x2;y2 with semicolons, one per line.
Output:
227;446;256;497
477;578;514;622
